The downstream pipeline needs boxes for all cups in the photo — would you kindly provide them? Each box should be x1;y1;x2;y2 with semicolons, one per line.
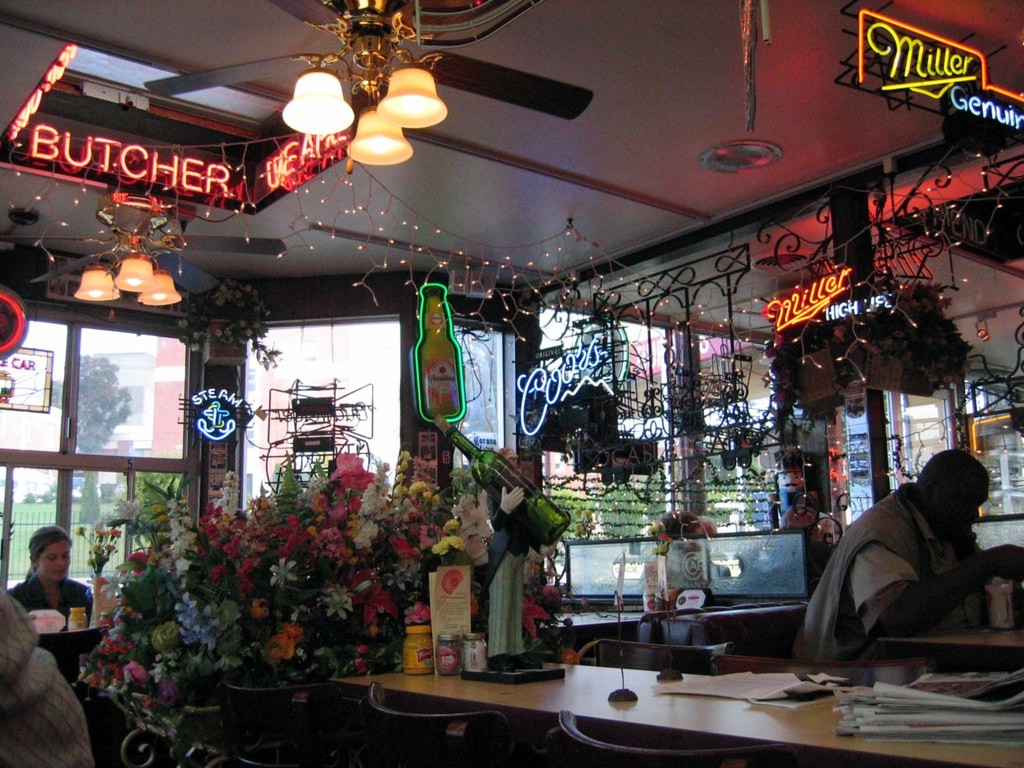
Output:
984;583;1014;628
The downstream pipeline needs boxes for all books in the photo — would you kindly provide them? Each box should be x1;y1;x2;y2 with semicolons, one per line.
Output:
828;666;1024;749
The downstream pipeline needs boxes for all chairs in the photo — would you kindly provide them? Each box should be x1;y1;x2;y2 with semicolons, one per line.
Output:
216;642;799;768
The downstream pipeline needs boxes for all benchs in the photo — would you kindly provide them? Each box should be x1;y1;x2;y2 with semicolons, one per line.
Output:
636;601;933;687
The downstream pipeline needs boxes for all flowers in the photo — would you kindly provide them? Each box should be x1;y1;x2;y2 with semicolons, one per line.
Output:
73;453;596;732
650;513;717;559
177;278;283;370
763;283;978;452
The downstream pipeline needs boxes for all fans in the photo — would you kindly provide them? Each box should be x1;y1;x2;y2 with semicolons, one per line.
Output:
143;0;596;147
28;195;288;296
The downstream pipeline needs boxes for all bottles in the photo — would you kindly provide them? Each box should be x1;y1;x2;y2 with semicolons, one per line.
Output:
432;414;572;547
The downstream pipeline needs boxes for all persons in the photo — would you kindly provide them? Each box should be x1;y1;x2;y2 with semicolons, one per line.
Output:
781;504;832;542
479;447;557;671
794;449;1024;661
0;588;97;768
6;525;176;768
641;511;718;594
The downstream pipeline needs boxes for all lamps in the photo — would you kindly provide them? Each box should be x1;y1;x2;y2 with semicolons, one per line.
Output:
282;51;448;166
975;318;990;343
71;244;183;307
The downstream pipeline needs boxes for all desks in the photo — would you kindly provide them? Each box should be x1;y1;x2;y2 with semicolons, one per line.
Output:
876;629;1024;673
340;664;1024;768
540;612;644;642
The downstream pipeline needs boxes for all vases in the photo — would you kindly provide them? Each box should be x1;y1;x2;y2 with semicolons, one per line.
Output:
863;343;935;398
793;340;847;417
